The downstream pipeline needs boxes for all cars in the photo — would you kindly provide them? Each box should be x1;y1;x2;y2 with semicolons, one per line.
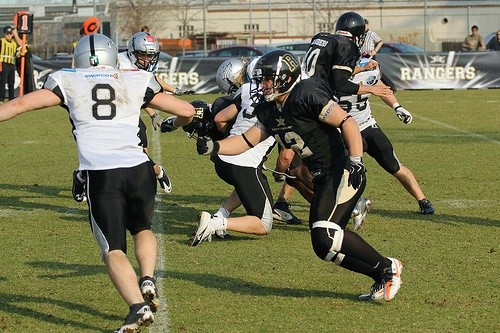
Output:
378;41;425;53
462;32;500;52
0;40;313;74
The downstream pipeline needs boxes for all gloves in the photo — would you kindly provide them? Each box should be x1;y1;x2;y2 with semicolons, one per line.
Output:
348;156;366;190
160;116;178;133
151;113;164;130
395;105;412;125
196;136;219;156
174;86;195;96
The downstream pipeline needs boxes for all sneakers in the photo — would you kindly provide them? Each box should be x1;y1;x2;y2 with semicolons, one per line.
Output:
157;166;172;193
72;170;84;202
139;276;159;313
418;198;435;214
191;211;213;246
360;282;385;301
272;203;302;224
114;298;155;333
211;213;226;239
352;198;372;231
384;257;403;302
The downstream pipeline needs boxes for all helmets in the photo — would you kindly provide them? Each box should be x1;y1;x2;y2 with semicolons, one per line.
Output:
335;12;366;38
128;32;159;65
215;59;244;94
74;34;117;68
183;99;213;132
247;56;262;83
3;26;12;33
254;50;301;94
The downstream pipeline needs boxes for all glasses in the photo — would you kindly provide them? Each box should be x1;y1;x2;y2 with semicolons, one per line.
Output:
6;33;11;35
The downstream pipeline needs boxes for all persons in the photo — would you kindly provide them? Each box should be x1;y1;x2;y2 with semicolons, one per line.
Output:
72;11;436;305
0;33;196;333
0;25;18;105
486;30;500;51
461;25;486;52
